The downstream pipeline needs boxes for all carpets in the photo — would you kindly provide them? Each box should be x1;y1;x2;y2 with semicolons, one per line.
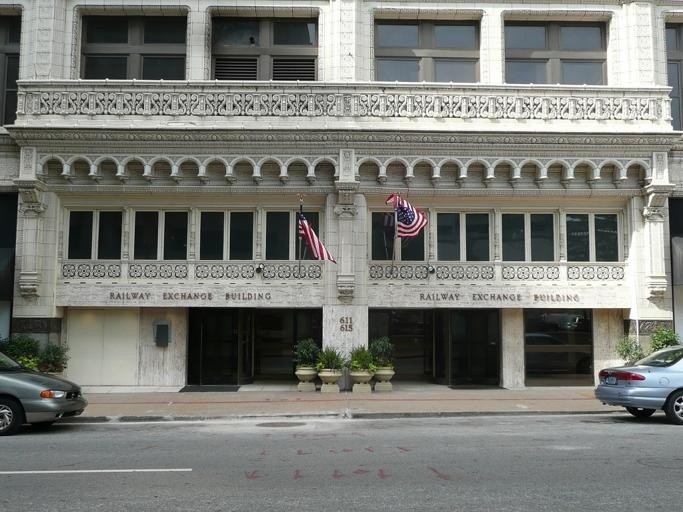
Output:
446;382;508;391
178;384;242;393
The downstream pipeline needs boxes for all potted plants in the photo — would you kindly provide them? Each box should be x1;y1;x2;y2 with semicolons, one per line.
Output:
368;335;397;392
39;342;70;380
343;346;377;396
292;338;320;393
316;345;348;394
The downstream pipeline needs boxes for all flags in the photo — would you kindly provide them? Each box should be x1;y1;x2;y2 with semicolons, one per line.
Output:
385;192;429;239
297;210;338;265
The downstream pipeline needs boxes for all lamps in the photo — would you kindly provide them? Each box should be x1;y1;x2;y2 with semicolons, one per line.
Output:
426;264;436;275
253;263;266;273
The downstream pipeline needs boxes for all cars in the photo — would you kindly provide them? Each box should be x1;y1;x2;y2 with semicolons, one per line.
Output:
543;310;588;335
596;345;683;427
524;333;594;374
0;351;87;435
